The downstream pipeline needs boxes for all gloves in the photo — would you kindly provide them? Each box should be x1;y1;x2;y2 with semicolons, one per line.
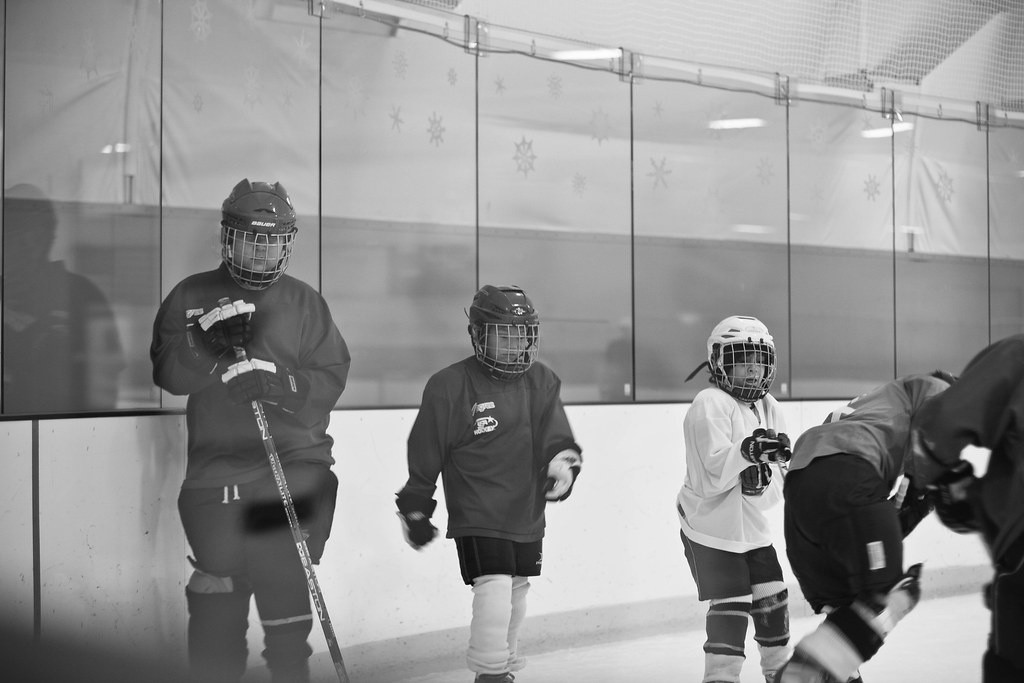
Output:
739;465;772;496
396;510;439;550
221;359;310;416
195;300;256;355
937;458;982;535
740;434;791;465
896;478;939;535
538;449;581;502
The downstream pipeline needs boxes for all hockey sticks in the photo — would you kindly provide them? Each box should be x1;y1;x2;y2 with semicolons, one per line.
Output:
764;428;790;479
217;295;350;683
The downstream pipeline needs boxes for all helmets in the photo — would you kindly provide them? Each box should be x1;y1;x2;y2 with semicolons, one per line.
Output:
686;315;776;402
463;284;540;383
220;178;297;291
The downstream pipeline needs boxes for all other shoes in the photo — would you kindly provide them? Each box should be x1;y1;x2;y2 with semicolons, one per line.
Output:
474;672;515;683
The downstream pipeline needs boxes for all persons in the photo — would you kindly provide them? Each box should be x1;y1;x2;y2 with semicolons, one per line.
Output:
771;370;960;683
150;177;351;679
912;334;1024;683
675;317;792;683
394;283;584;683
5;183;128;414
